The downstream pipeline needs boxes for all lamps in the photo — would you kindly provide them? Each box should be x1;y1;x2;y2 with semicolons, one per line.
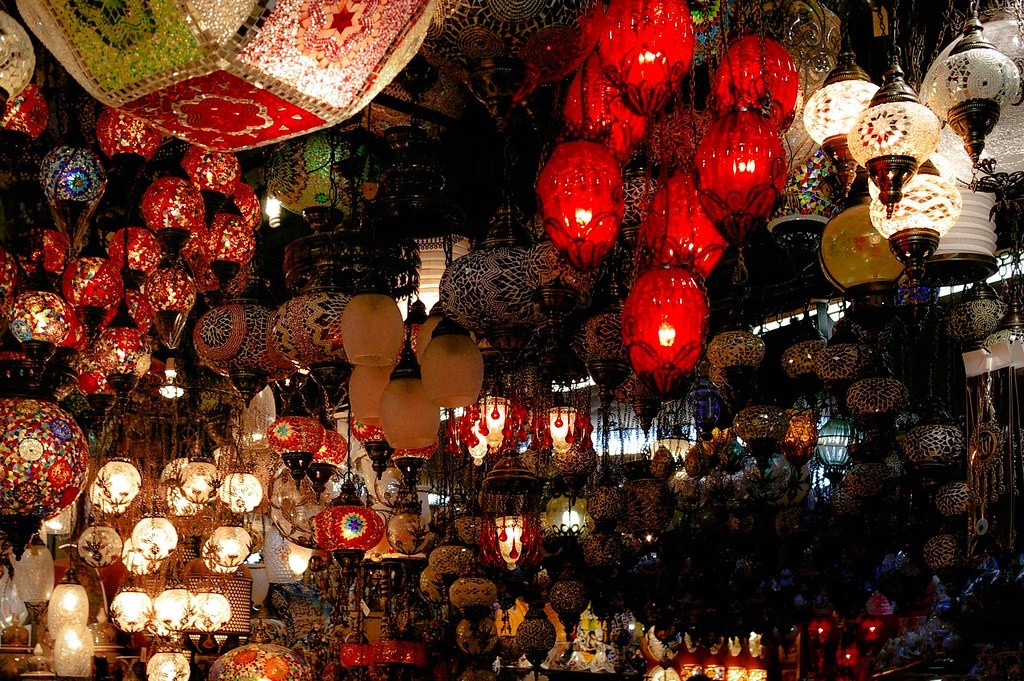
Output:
340;49;485;453
804;0;1024;291
